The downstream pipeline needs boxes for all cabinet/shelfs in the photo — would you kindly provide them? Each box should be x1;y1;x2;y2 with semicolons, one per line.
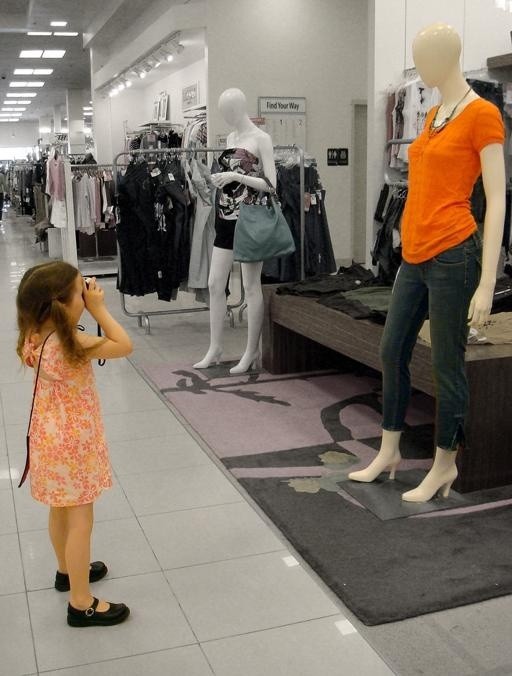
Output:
262;273;512;493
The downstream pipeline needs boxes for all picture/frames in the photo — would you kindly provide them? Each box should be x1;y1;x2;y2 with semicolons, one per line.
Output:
179;79;200;113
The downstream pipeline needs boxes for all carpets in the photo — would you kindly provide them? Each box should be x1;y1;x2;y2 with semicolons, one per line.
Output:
121;335;512;625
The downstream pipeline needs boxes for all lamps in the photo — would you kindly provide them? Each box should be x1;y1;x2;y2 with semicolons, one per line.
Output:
94;33;182;98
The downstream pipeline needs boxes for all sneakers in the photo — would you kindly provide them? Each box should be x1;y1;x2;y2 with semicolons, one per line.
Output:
53;559;108;594
62;596;131;628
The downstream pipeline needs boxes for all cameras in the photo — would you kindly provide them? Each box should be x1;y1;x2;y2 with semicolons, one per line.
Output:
84;276;97;291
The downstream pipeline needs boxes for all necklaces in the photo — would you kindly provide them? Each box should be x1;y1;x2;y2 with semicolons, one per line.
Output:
431;86;474;129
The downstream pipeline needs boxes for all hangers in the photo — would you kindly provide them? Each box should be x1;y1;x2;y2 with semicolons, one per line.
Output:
12;144;309;178
397;67;421;89
385;182;408;197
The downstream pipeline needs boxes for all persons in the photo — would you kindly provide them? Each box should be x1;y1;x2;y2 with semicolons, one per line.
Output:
347;22;507;503
191;87;277;374
15;260;134;627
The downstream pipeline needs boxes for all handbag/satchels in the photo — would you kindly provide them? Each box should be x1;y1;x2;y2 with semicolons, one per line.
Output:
231;175;296;266
